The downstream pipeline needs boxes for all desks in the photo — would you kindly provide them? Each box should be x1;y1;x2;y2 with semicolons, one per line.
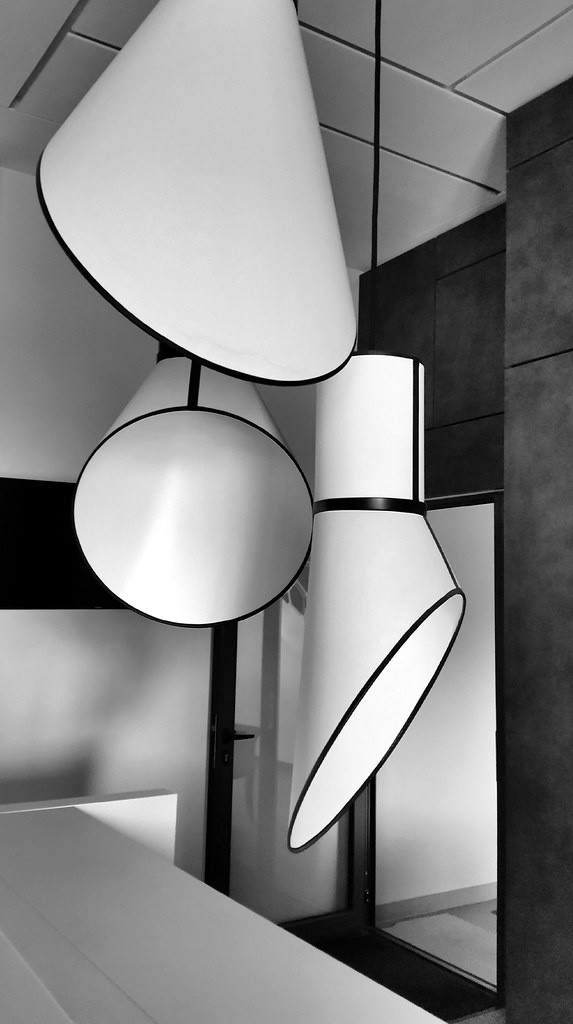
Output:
0;790;448;1024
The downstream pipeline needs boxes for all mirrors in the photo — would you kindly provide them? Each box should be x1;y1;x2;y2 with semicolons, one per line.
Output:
370;493;500;997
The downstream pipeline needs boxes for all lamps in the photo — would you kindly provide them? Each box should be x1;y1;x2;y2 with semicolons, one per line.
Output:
70;342;314;627
287;0;465;854
36;0;359;387
